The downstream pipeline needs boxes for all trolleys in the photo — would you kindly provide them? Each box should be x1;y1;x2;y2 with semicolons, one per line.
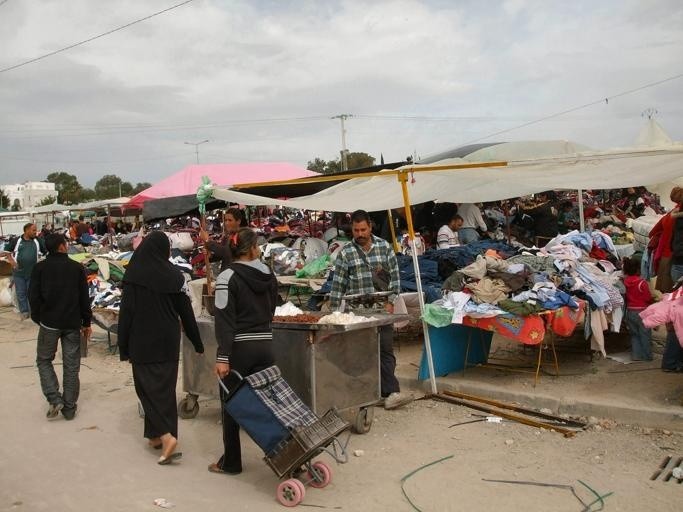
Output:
178;300;410;447
211;366;349;507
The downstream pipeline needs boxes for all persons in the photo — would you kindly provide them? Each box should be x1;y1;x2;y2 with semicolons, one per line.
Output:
117;232;205;466
143;205;353;282
1;223;56;258
622;187;683;371
331;211;416;409
10;224;40;320
368;186;656;251
208;228;277;475
63;216;142;247
26;233;92;420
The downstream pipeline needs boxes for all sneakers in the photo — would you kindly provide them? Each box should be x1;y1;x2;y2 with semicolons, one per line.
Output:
208;463;236;473
47;403;64;418
384;392;414;410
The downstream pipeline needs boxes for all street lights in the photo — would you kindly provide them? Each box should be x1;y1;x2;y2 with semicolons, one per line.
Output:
183;138;208;165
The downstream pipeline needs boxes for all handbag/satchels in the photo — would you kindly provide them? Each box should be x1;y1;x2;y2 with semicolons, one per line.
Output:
372;268;391;291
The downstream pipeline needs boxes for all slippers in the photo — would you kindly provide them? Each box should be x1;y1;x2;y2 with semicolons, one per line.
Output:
154;443;182;465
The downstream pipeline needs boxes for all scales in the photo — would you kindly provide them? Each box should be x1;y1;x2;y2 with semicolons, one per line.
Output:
340;291;395;318
